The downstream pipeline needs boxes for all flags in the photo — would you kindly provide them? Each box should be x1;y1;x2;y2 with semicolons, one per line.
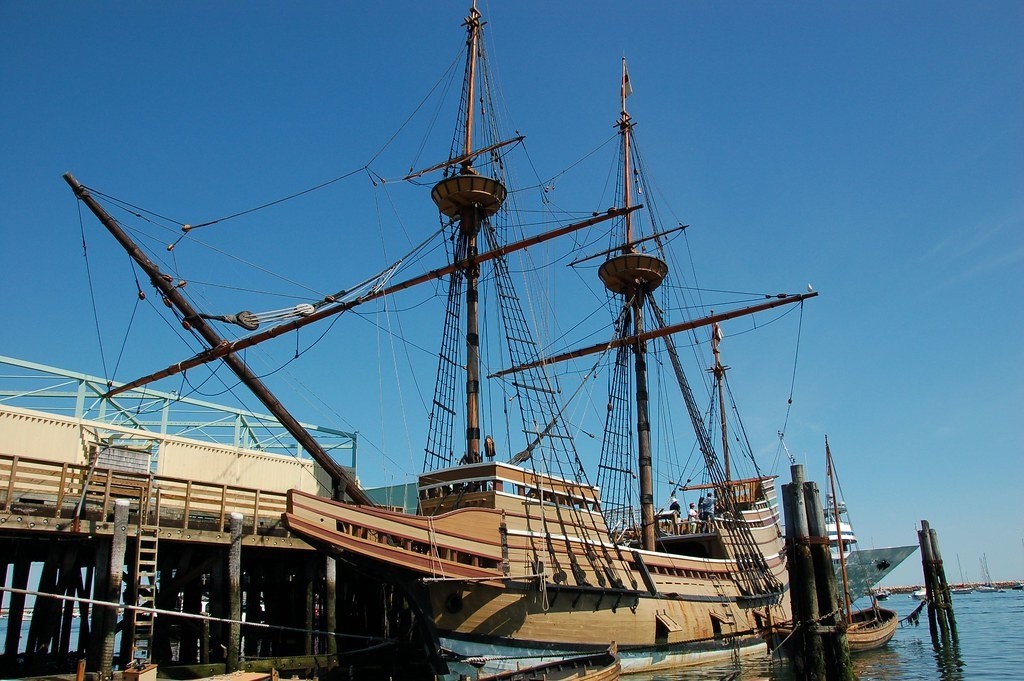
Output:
711;323;724;347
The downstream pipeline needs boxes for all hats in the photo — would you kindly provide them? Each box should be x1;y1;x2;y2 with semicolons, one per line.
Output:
689;503;695;507
671;498;678;504
699;497;704;502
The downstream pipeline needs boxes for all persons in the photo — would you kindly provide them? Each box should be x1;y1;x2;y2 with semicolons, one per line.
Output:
671;498;681;523
688;493;718;533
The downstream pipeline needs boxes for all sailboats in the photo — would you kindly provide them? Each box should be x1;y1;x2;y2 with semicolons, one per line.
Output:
752;436;899;659
62;4;923;681
869;553;1024;600
